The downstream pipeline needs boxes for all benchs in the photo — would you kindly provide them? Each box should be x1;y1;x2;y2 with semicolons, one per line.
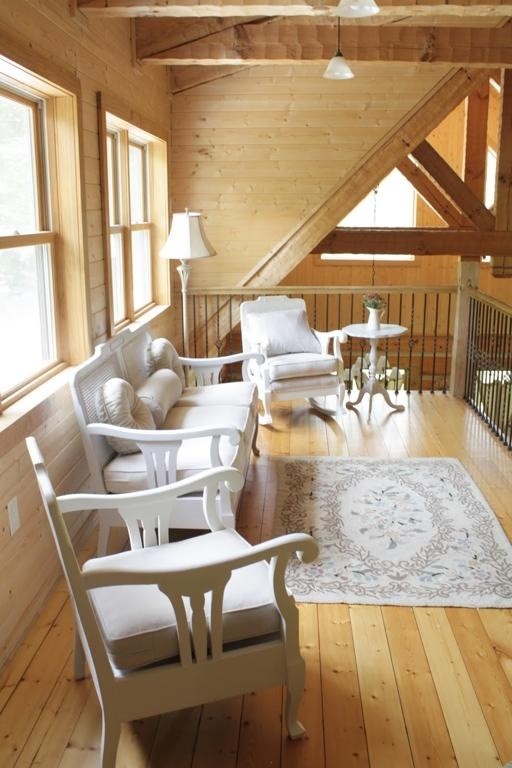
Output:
70;322;265;557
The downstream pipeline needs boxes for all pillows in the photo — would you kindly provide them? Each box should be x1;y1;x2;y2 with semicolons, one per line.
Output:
96;378;157;455
145;337;185;392
246;310;321;356
135;368;182;428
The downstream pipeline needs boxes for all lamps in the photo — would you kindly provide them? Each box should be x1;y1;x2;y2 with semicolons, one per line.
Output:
334;0;379;18
158;207;216;387
321;16;356;79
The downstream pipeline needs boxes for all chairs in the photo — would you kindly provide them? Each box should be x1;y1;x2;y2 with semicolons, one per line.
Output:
240;295;347;425
26;436;318;768
55;584;58;586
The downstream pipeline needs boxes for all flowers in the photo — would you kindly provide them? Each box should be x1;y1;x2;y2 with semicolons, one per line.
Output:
362;292;387;309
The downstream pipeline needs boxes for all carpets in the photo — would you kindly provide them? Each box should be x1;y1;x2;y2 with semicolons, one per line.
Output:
272;456;512;609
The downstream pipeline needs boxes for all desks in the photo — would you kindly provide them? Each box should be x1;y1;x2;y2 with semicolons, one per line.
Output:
342;323;408;418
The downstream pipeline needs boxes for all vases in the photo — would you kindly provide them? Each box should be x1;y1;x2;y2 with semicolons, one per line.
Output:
366;306;384;330
